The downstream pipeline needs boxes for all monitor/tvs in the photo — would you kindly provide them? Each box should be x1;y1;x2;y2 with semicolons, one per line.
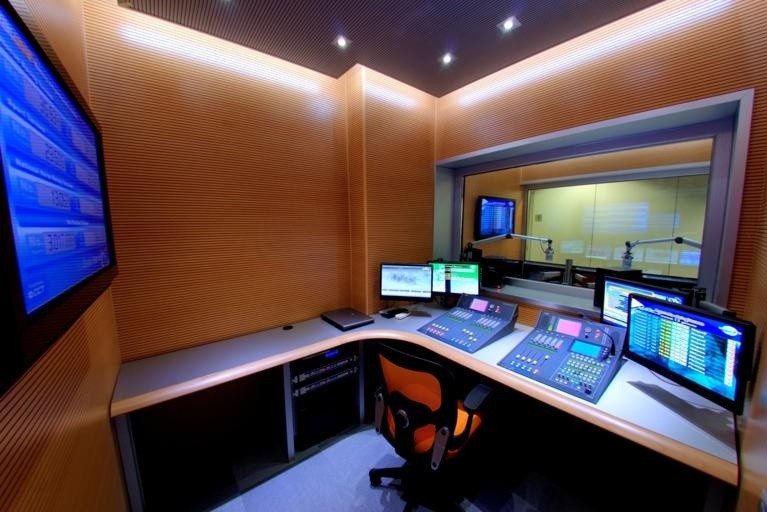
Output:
577;312;615;355
474;195;516;241
0;1;119;394
624;293;757;416
380;264;433;300
428;262;479;294
600;276;691;327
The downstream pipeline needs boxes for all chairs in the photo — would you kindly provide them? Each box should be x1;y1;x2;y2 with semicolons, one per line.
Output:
368;342;492;512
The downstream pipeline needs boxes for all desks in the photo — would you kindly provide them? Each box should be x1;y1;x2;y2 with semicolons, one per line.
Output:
110;302;740;512
